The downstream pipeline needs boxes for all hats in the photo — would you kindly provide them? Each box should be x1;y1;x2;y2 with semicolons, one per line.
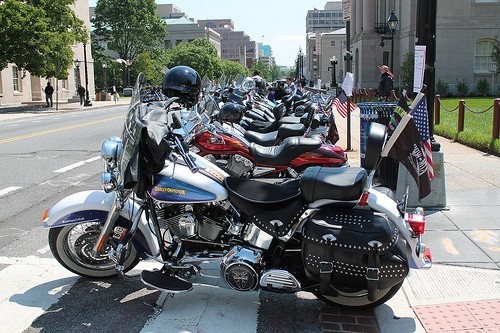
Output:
377;65;395;79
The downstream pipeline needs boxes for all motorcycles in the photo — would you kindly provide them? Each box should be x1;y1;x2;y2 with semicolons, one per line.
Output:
43;66;432;311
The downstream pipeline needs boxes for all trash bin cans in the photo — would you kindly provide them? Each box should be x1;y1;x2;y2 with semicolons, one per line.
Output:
357;101;398;168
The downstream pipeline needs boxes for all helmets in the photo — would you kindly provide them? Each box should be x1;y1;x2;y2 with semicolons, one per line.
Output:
295;104;306;116
256;80;261;87
162;66;201;106
219;104;243;122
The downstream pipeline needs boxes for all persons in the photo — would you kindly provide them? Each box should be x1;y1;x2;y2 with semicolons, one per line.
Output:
78;86;85;105
275;81;287;100
377;65;395;103
252;70;266;96
45;82;54;108
111;89;119;101
286;78;295;91
301;80;306;88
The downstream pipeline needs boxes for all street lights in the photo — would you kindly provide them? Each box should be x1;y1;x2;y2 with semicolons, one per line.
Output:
329;56;338;88
294;45;304;85
380;11;399;89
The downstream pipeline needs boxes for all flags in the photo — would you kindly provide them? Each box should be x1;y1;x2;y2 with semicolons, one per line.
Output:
410;95;434;181
385;118;431;203
333;91;356;118
387;95;410;135
